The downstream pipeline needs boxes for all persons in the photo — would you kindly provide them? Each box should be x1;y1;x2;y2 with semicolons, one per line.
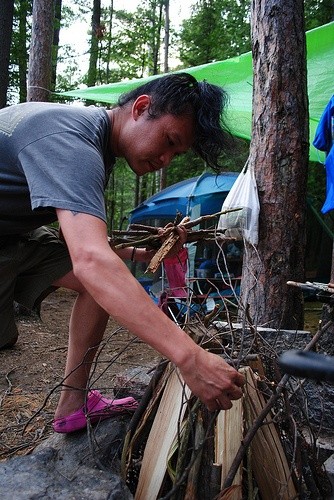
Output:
0;70;247;439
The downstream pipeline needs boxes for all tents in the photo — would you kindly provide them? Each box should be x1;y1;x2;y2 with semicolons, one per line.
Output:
119;168;246;297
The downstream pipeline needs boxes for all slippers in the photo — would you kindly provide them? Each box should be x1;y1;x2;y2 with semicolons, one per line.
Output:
54;389;139;433
